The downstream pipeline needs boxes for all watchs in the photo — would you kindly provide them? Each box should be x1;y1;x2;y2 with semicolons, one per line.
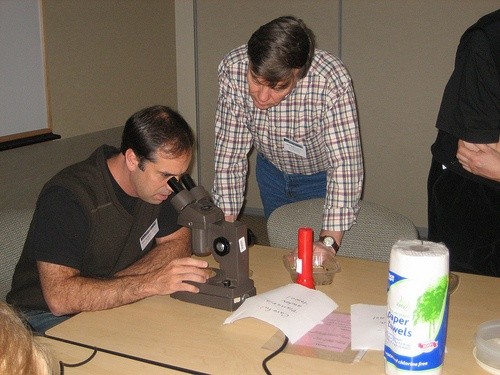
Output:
320;235;339;253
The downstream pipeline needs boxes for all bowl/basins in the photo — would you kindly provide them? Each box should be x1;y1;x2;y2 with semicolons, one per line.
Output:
473;320;500;368
282;251;339;287
448;272;460;295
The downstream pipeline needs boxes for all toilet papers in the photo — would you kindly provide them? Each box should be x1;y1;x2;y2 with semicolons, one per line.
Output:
383;239;450;375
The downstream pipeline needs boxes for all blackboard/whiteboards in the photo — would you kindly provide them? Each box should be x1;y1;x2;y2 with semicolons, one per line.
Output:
0;0;52;143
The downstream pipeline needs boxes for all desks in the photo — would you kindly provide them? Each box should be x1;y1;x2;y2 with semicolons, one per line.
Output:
34;245;500;375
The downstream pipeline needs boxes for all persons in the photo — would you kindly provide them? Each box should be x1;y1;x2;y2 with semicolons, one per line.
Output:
427;9;500;277
6;105;210;334
211;16;364;264
0;302;53;375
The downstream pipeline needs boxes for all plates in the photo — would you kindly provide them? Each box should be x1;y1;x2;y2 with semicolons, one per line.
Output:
473;347;500;374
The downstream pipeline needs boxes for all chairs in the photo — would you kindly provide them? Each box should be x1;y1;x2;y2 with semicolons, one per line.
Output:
267;199;419;262
0;126;123;303
